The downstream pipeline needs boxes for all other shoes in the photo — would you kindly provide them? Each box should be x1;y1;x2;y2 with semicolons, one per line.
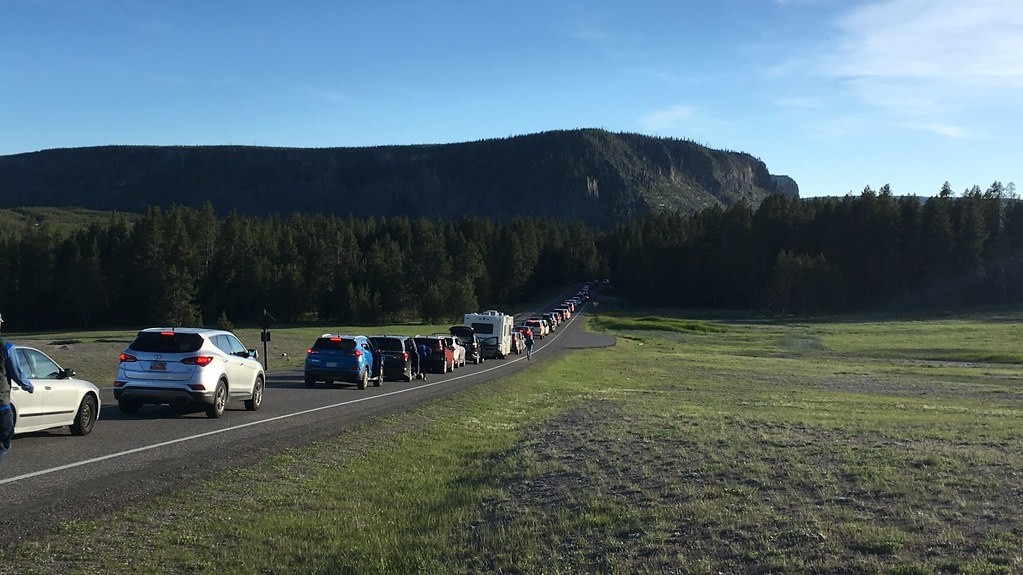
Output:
528;359;530;360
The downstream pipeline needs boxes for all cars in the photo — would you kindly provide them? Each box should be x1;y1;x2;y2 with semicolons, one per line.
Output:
7;346;102;438
544;278;609;333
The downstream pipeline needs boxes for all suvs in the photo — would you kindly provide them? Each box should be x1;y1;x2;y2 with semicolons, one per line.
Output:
445;337;466;368
305;333;383;390
114;327;266;419
510;331;526;354
369;334;421;383
515;326;533;339
414;334;454;374
448;326;499;364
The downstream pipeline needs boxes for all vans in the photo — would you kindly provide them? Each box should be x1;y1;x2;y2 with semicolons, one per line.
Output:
524;320;549;339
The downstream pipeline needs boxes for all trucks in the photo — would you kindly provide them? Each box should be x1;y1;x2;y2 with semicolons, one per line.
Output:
464;310;515;359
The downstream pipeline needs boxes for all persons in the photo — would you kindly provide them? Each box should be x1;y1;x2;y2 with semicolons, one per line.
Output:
0;313;34;459
417;345;427;381
525;335;533;360
